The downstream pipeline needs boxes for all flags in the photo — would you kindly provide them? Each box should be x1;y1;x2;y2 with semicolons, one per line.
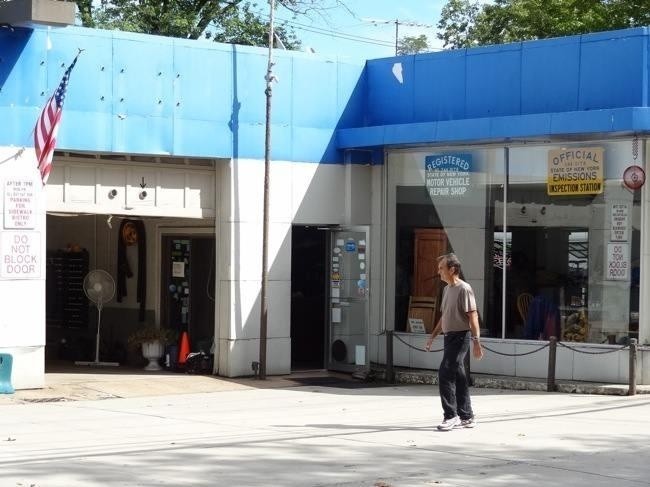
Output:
33;52;83;188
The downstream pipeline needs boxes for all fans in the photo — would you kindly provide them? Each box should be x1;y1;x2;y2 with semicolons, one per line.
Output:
74;269;119;367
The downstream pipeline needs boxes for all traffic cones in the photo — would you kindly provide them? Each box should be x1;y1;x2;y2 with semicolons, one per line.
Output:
178;331;191;363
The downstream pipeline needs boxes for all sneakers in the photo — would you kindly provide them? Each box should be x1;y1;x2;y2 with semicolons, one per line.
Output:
452;418;475;429
437;415;461;431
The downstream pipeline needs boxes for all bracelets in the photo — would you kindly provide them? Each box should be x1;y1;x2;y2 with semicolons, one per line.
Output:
470;335;480;341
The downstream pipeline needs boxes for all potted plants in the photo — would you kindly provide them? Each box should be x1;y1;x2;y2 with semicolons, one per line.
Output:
127;322;175;371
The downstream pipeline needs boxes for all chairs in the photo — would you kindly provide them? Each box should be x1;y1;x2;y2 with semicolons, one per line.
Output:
406;295;438;334
517;293;535;329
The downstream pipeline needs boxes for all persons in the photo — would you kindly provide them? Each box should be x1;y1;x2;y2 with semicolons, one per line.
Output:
424;253;484;431
